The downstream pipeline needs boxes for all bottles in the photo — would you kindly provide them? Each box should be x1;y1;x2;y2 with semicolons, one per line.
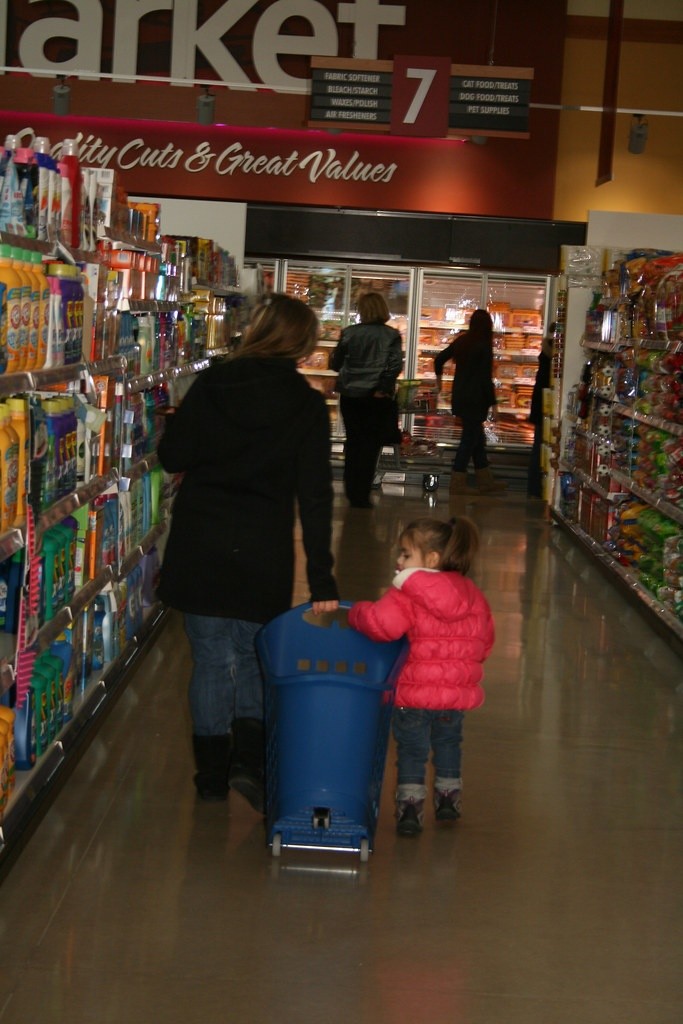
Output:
399;428;411;457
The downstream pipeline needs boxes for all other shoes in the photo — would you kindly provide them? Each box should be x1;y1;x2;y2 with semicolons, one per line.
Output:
527;493;543;502
349;496;374;509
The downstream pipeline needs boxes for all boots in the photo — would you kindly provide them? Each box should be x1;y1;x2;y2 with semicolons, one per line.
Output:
233;715;270;813
477;467;509;493
449;470;478;495
191;732;236;802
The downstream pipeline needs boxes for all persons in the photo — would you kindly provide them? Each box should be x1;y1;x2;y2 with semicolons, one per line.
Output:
327;292;403;509
432;308;507;495
157;293;340;815
526;323;556;495
346;513;496;837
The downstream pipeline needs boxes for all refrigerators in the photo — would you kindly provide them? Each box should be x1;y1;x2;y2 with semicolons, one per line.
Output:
243;255;553;476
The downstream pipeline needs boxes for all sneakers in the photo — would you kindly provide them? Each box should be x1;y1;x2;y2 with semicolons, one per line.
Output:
434;788;461;821
393;794;426;837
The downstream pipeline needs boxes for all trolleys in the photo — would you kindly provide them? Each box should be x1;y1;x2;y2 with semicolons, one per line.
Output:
371;389;466;493
250;599;414;863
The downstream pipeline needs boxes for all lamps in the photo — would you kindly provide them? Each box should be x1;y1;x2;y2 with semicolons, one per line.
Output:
629;114;648;154
196;88;218;125
50;76;71;114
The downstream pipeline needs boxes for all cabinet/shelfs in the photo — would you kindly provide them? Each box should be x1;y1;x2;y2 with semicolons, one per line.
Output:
0;235;248;891
552;343;683;640
